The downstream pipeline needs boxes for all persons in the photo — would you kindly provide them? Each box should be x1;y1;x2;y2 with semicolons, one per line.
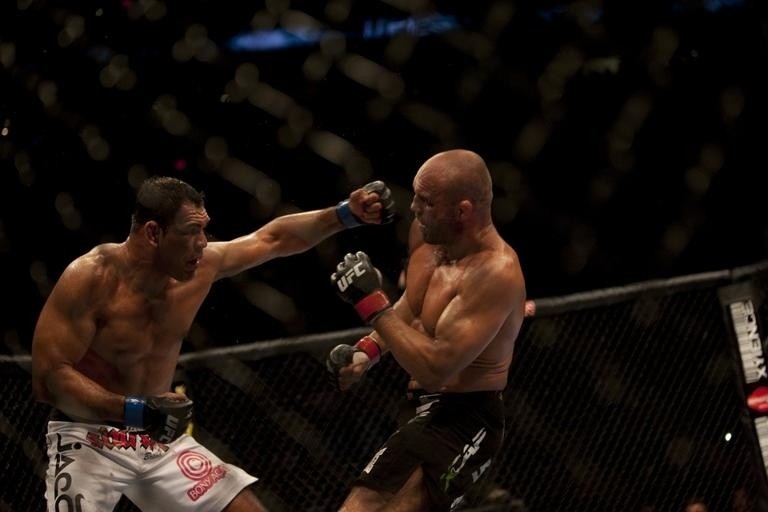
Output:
30;177;397;512
326;149;526;512
